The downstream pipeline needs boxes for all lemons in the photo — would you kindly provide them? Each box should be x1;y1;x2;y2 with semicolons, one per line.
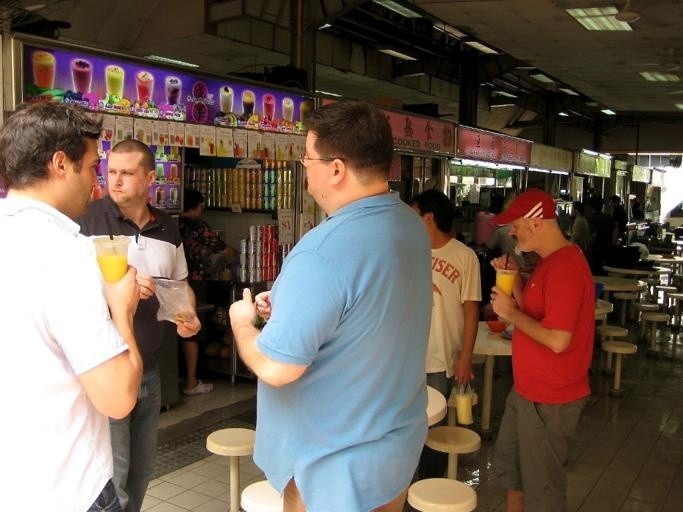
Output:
118;99;130;107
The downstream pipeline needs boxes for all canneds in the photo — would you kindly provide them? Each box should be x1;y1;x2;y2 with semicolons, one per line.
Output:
185;168;206;209
239;254;246;268
248;225;257;242
232;169;262;210
248;254;256;270
263;161;293;211
256;224;292;282
247;240;257;254
206;168;232;207
248;269;256;283
240;268;247;283
240;239;247;254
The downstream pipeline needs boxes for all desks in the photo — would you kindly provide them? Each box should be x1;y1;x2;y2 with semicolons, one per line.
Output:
425;383;448;429
473;320;511;439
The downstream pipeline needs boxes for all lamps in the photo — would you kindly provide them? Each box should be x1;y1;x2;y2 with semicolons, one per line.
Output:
614;1;640;25
659;46;682;75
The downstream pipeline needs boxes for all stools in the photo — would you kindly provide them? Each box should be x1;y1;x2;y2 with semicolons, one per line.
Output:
592;244;682;399
204;426;256;512
240;481;284;512
406;477;478;512
424;426;481;482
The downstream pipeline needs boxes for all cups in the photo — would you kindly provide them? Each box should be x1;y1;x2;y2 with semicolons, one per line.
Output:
219;85;233;112
164;77;181;105
493;267;518;297
94;236;131;282
169;188;178;204
32;51;55;89
156;163;164;179
155;188;165;204
135;70;154;106
70;58;92;94
105;64;124;99
455;395;473;425
282;97;293;120
241;91;254;115
169;164;177;178
262;93;275;119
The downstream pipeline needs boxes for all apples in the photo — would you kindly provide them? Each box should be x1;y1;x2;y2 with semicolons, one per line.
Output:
109;94;120;104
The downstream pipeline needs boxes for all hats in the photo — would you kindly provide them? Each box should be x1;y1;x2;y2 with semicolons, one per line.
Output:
490;188;556;225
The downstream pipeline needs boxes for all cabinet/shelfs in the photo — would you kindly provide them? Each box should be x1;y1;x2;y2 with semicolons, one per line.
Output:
188;280;268;386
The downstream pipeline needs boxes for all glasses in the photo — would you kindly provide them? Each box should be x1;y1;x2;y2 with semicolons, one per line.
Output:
297;151;348;165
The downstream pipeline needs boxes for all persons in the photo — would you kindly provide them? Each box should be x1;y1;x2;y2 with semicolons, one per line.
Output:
229;97;431;512
452;185;664;306
182;187;241;394
1;101;144;512
410;189;483;482
490;187;595;512
70;138;203;511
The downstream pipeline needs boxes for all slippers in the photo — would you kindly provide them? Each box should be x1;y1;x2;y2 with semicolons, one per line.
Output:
183;379;213;395
